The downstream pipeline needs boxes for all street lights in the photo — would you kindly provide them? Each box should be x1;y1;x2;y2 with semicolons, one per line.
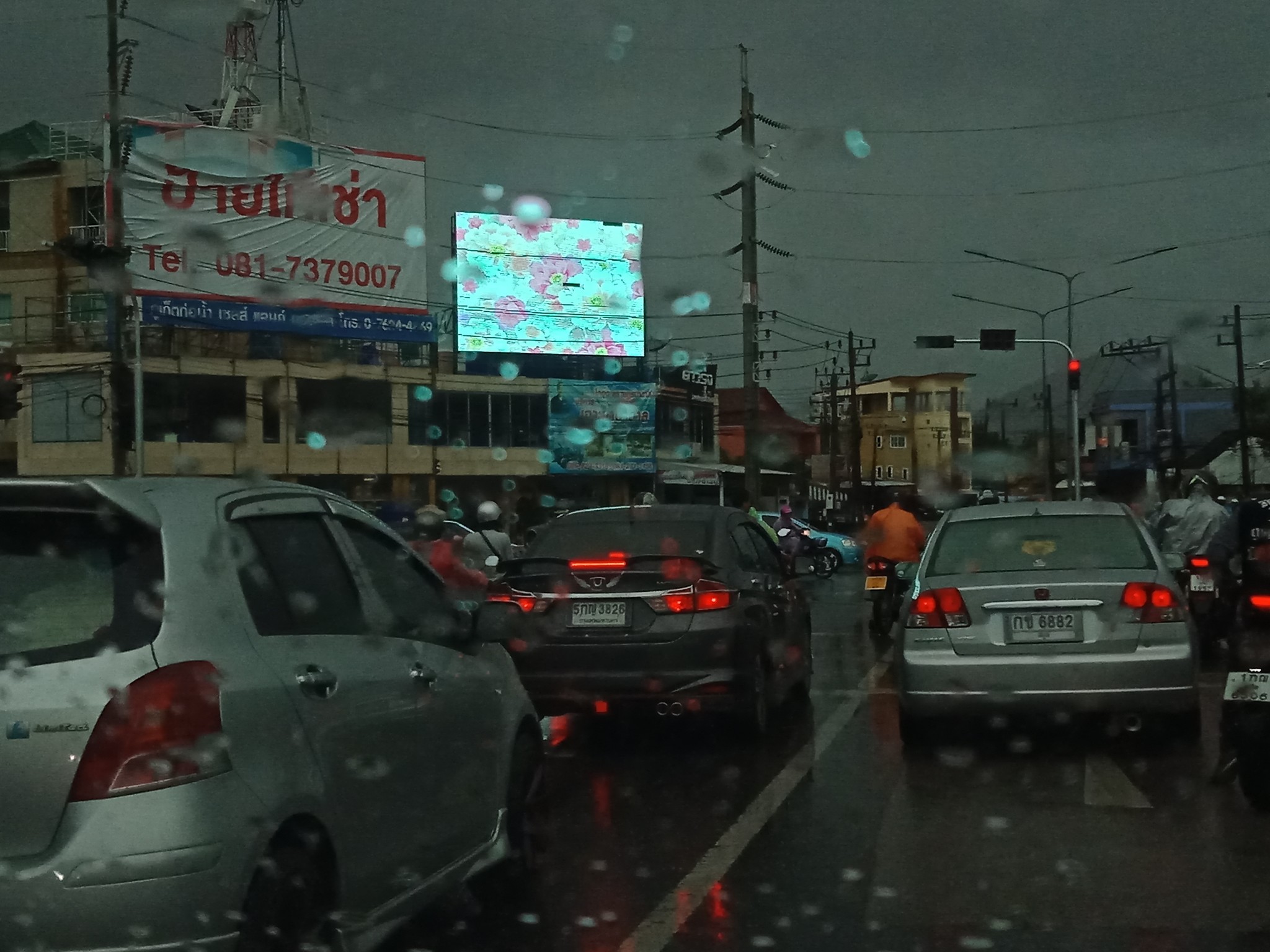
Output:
952;286;1138;503
963;246;1183;510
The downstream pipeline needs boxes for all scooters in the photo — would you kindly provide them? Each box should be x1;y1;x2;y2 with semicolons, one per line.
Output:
865;555;901;637
1157;512;1239;659
1222;522;1269;808
777;529;837;578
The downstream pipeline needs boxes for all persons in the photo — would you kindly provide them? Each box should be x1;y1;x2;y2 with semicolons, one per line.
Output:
976;484;1000;506
1146;464;1270;635
747;504;811;579
400;500;517;616
633;492;659;507
860;488;928;642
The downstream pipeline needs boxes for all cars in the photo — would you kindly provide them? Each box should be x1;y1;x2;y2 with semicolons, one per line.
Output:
897;504;1198;741
750;510;863;579
0;475;553;952
476;504;821;747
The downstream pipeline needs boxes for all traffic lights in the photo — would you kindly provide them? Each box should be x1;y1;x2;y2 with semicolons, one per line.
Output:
0;353;31;423
1069;359;1087;398
